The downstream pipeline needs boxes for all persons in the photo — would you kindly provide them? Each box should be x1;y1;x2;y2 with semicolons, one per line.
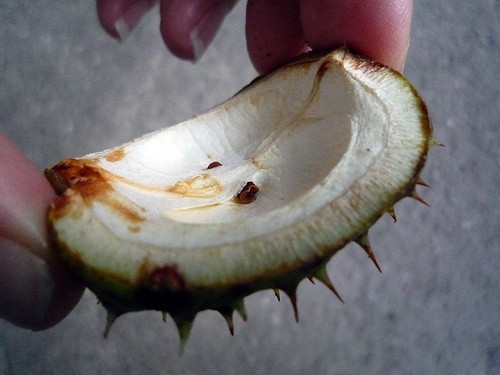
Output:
0;0;416;338
